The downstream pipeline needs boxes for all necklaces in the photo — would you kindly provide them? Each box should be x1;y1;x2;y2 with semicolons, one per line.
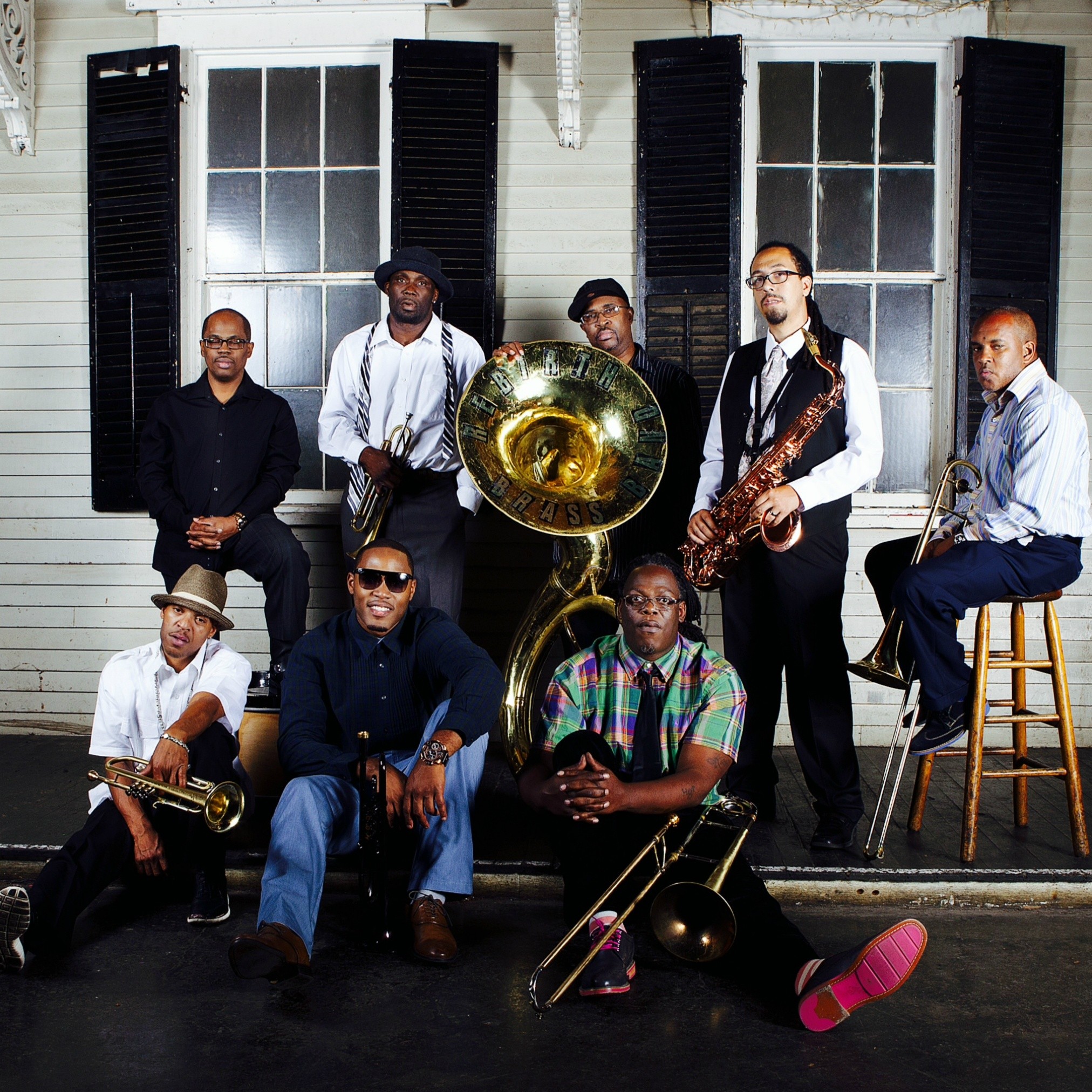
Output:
155;672;195;731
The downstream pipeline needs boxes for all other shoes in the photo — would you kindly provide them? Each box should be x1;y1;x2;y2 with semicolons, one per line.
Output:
907;701;969;755
269;655;290;696
903;703;927;730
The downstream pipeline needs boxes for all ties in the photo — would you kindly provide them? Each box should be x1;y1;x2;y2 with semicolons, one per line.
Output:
629;669;664;783
737;346;786;482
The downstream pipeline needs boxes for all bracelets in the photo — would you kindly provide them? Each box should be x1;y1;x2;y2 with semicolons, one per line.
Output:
160;733;190;752
954;531;966;544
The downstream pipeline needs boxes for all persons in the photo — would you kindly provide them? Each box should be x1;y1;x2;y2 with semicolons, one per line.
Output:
317;247;487;629
511;557;928;1034
864;307;1092;755
137;308;312;708
687;240;882;850
228;537;508;981
0;564;252;973
492;278;701;660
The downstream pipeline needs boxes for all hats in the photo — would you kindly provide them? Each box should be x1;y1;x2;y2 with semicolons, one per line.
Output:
374;246;454;304
567;278;630;322
150;563;234;631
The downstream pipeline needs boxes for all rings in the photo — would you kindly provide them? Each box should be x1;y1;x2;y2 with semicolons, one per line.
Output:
770;511;777;517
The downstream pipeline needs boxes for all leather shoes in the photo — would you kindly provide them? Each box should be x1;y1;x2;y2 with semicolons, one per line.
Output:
185;870;230;925
811;814;858;848
406;889;459;963
227;922;315;991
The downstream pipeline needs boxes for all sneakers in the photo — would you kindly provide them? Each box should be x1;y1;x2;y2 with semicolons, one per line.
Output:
798;920;929;1032
0;884;33;973
578;920;637;996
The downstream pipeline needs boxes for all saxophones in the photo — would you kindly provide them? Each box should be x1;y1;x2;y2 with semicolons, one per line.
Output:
676;326;847;594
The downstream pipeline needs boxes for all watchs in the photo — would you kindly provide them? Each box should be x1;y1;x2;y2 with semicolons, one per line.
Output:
421;740;449;767
231;514;247;531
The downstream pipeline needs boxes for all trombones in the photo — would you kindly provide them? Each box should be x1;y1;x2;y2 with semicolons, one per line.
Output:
526;791;758;1022
845;461;984;861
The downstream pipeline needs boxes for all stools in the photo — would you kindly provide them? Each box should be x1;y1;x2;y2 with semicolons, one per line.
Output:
908;590;1089;863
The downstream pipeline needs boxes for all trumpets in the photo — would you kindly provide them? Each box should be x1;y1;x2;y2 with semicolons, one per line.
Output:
347;414;423;562
85;757;246;833
355;727;396;957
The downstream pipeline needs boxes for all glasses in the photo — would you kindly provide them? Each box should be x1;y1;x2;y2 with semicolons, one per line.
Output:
746;270;805;289
203;337;249;349
580;305;630;326
352;568;414;593
617;593;682;610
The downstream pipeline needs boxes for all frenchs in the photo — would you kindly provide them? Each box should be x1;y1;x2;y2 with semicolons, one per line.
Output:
454;341;669;778
